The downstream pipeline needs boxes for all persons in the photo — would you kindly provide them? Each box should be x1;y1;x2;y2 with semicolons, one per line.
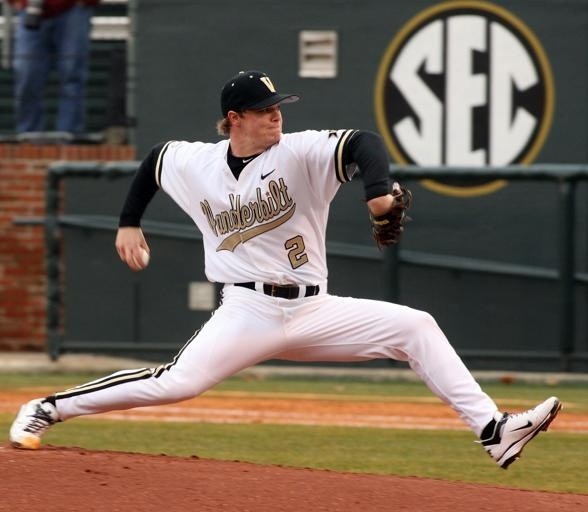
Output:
11;70;562;468
8;0;110;146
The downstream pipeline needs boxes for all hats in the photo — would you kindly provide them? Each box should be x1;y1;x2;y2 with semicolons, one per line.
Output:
220;71;300;118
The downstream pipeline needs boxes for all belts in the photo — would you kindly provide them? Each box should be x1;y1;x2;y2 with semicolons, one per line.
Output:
234;282;319;300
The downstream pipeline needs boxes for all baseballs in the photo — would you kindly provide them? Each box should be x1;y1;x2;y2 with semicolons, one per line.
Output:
132;248;150;268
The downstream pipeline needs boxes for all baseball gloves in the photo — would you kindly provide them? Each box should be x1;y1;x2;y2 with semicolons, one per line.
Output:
361;181;412;252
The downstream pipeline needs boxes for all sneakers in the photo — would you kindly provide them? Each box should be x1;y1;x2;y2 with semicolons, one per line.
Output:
475;397;563;469
8;397;62;450
